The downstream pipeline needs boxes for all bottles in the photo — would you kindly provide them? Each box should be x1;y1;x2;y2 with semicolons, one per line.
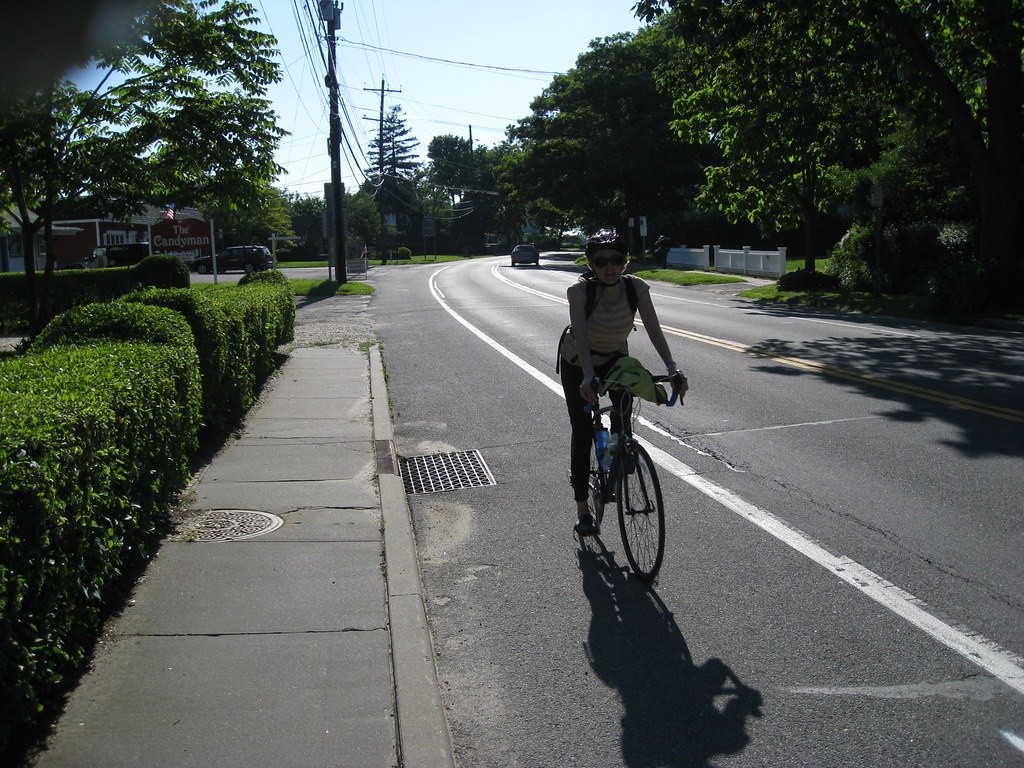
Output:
601;433;618;469
595;427;610;467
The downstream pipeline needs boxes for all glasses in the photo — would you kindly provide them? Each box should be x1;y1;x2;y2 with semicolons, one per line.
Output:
593;254;625;267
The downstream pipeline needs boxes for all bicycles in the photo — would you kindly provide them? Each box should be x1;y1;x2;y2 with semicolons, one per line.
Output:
566;368;688;581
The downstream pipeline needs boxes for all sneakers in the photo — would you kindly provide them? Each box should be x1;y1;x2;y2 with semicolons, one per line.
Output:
610;450;637;474
573;513;594;542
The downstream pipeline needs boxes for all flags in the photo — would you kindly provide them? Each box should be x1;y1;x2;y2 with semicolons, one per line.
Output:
162;204;175;220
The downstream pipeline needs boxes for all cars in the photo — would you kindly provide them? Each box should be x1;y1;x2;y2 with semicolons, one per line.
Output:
511;244;540;267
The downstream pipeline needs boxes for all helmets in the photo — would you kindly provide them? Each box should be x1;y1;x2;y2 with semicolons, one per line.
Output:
585;228;628;259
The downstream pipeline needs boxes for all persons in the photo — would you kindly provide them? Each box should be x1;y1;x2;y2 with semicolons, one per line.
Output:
556;228;689;533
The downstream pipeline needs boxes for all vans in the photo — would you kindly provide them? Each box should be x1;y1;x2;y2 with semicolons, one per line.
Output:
67;242;151;269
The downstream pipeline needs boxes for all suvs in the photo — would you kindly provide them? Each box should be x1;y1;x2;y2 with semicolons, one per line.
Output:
192;244;278;275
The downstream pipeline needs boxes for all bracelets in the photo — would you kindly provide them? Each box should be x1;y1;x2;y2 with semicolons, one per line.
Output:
667;362;676;371
